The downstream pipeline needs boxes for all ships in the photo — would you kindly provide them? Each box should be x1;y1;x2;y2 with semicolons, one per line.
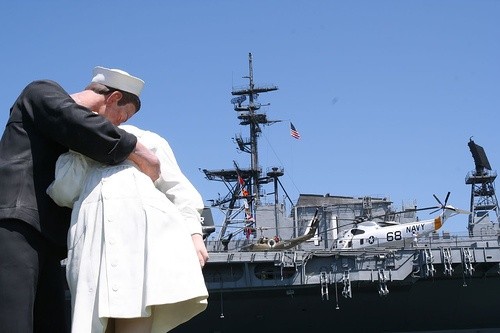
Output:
191;50;500;333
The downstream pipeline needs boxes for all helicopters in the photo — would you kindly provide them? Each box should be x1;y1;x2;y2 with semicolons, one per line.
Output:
309;192;472;253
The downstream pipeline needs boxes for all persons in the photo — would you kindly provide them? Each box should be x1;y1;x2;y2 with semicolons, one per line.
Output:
46;120;212;332
0;63;161;333
274;235;280;242
410;230;420;246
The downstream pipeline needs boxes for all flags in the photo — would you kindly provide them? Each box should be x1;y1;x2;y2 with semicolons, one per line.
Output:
237;176;255;241
286;122;300;138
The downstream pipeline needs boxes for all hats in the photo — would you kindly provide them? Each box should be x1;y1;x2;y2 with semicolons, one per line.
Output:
92;65;146;97
